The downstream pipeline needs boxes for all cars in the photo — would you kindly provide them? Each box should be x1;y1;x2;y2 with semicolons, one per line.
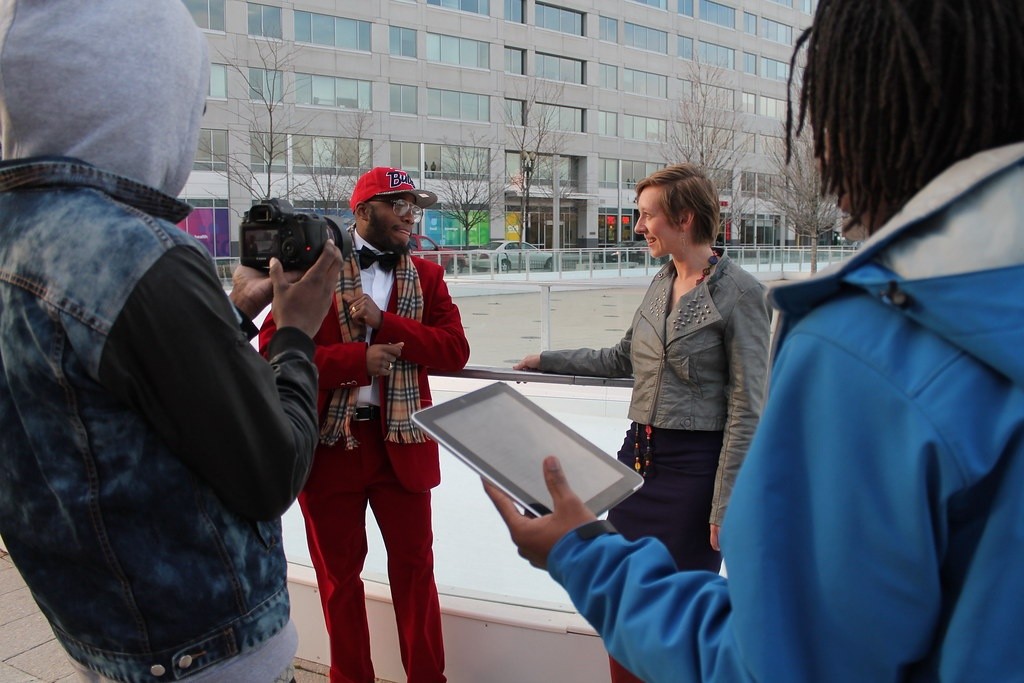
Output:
833;240;862;256
608;239;669;266
408;233;466;274
465;240;553;272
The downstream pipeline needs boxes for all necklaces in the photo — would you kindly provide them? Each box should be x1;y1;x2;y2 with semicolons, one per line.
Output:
696;251;719;287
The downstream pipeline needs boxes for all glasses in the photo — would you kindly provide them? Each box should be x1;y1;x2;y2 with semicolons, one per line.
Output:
367;199;423;223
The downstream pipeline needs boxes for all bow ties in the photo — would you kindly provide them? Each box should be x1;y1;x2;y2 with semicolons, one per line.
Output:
359;245;400;273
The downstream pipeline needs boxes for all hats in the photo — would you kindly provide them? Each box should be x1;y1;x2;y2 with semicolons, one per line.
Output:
350;166;438;215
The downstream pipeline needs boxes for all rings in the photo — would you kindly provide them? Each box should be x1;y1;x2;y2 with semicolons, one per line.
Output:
388;363;394;370
352;306;356;312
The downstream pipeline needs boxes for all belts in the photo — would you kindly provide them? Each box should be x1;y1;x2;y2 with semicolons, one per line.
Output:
351;406;379;421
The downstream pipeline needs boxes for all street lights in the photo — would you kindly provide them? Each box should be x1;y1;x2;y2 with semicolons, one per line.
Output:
521;149;535;243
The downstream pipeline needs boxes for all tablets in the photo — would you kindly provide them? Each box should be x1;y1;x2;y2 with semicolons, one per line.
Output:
410;381;644;520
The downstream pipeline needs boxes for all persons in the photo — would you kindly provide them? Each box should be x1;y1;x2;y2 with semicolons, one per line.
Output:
0;0;343;683
513;164;774;683
258;166;470;683
479;0;1024;683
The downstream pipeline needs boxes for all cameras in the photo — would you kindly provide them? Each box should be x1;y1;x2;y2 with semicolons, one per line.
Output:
238;197;352;275
